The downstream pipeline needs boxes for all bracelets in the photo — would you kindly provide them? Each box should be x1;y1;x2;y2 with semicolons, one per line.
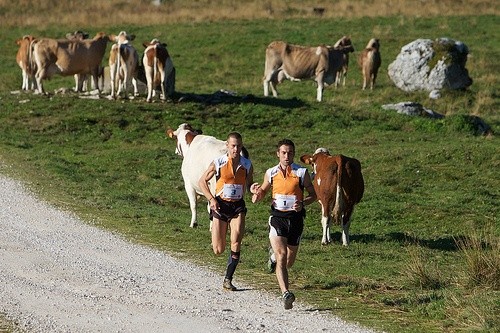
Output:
209;196;215;202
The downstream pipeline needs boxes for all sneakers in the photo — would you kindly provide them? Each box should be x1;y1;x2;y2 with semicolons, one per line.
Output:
268;256;276;273
223;278;236;291
284;292;294;310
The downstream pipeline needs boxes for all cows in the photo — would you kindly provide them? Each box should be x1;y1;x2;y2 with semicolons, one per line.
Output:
261;41;341;102
335;36;353;88
299;148;363;246
166;121;249;231
361;37;382;92
16;29;176;102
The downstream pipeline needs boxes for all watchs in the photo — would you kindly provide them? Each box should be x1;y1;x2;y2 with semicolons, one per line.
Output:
301;200;307;206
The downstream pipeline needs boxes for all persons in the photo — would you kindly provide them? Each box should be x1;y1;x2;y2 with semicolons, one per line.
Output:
250;140;317;310
197;132;260;293
